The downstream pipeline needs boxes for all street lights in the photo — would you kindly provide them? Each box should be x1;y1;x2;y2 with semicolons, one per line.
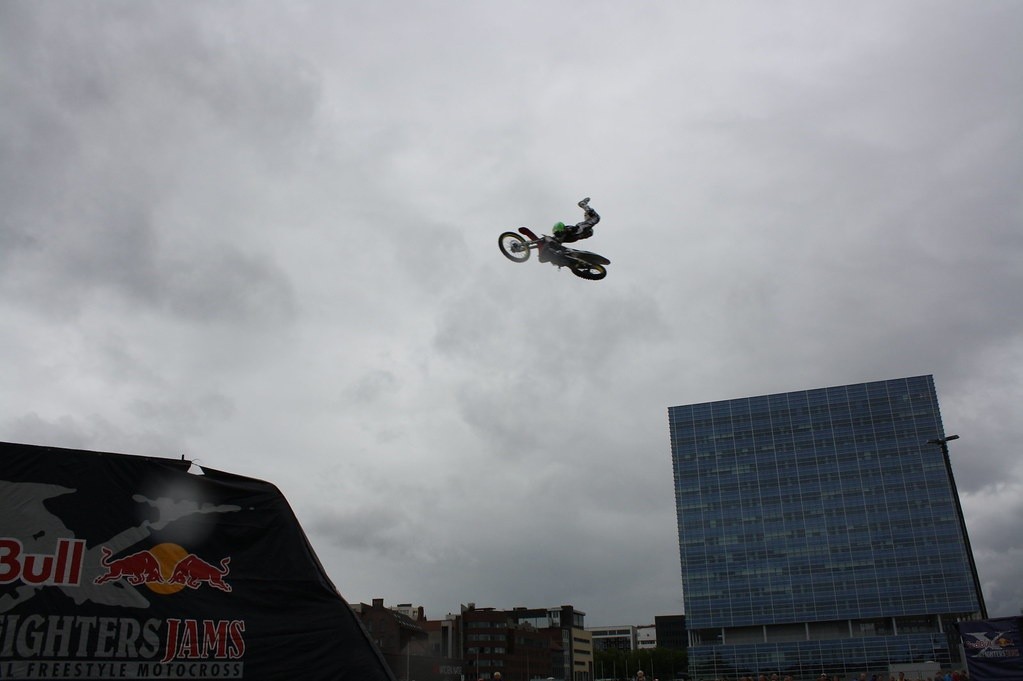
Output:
927;433;990;619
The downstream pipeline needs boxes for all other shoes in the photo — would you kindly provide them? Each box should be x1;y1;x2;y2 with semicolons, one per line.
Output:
578;197;590;207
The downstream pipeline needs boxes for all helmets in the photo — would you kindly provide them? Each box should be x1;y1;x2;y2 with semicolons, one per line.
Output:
553;222;566;234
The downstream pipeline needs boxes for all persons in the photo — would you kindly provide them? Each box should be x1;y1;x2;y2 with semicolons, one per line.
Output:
553;197;601;242
635;671;646;681
716;670;970;681
494;672;502;681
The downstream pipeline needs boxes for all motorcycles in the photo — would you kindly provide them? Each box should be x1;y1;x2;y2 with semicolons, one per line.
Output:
499;226;612;281
